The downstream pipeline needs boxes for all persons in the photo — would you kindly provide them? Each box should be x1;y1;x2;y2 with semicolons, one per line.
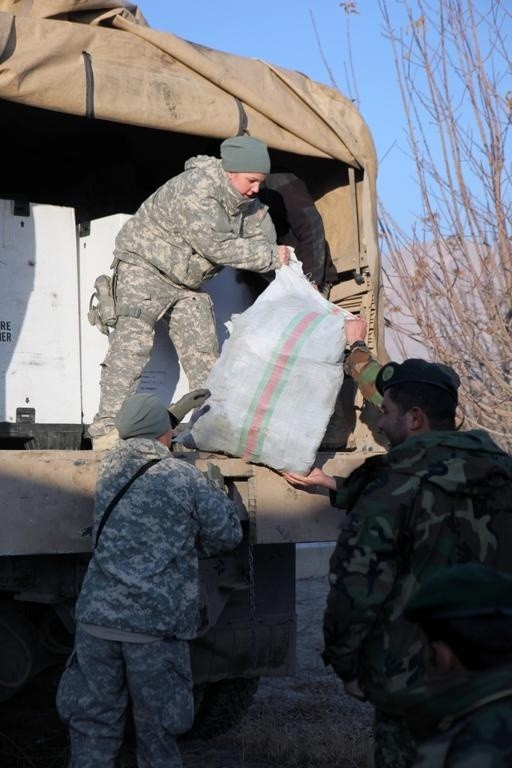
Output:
249;170;340;309
83;130;298;450
280;312;394;513
56;385;247;768
316;355;512;767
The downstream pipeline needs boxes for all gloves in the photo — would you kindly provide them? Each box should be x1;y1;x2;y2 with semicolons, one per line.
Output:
165;387;215;422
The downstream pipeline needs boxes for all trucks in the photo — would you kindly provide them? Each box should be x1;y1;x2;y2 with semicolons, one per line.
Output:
1;0;389;749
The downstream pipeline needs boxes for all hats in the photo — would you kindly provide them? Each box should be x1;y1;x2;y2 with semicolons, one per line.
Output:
113;392;174;441
373;357;464;399
218;136;271;176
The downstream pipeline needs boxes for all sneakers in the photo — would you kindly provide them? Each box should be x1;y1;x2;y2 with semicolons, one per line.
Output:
92;429;129;452
